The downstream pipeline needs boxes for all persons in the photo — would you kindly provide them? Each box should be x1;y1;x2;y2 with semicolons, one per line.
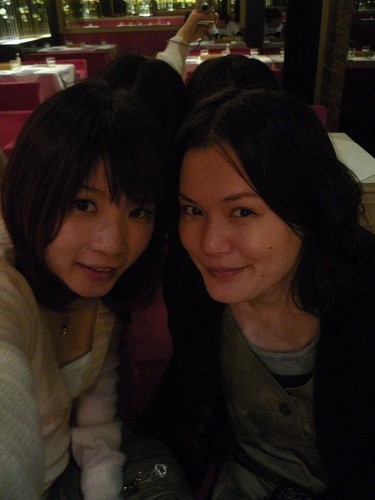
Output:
135;83;375;500
0;81;171;499
91;0;220;89
212;9;242;42
187;52;282;91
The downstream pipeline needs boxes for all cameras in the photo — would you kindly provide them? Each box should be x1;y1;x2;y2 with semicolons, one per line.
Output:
195;0;214;25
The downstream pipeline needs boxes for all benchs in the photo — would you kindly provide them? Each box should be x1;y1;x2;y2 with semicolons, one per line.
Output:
22;58;89;80
0;80;44;153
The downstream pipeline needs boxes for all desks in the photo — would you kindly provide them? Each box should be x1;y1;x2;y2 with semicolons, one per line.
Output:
36;44;118;77
327;129;375;233
346;50;375;68
0;63;76;110
188;40;245;50
185;50;284;76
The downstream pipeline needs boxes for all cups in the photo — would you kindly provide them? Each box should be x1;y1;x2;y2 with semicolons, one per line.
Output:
42;39;107;67
347;45;370;58
196;34;260;60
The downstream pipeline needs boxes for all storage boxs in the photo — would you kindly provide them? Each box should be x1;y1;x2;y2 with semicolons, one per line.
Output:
189;46;250;56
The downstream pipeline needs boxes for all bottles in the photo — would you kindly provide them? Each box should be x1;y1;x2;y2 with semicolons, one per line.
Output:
226;41;230;55
14;51;23;71
